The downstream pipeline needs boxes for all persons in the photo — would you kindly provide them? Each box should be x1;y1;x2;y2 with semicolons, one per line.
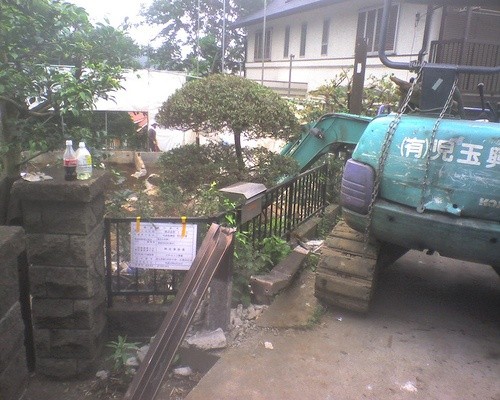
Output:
148;123;161;151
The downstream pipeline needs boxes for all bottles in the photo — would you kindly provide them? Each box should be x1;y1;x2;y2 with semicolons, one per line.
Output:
63;140;77;181
75;141;92;181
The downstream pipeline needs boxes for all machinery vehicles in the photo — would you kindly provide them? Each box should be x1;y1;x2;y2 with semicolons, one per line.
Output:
253;0;500;318
